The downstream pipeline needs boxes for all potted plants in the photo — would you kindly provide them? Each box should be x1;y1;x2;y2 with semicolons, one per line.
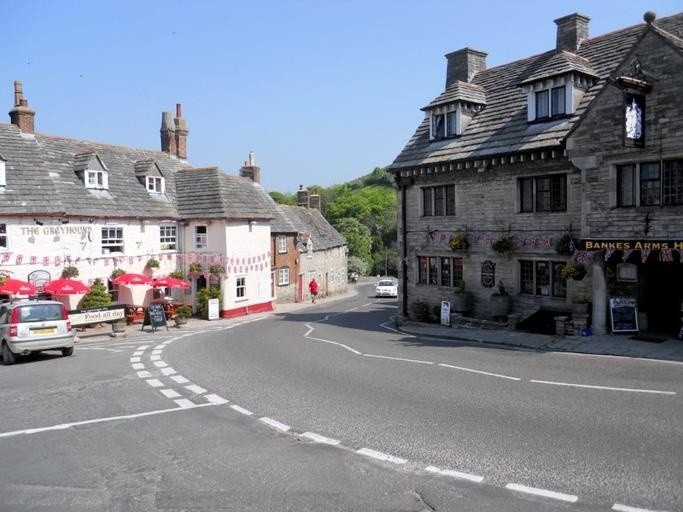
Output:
410;279;512;316
174;306;193;325
561;261;587;281
195;287;211;319
112;318;127;333
572;295;591;313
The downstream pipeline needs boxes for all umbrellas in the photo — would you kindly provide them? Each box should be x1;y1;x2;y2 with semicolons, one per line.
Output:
150;277;191;297
42;279;91;296
112;273;153;286
0;278;36;296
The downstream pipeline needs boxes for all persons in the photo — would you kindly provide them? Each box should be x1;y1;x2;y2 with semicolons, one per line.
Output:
309;278;319;303
348;271;359;283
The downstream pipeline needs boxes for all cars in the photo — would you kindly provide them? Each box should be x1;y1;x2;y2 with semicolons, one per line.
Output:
0;300;75;365
374;280;399;297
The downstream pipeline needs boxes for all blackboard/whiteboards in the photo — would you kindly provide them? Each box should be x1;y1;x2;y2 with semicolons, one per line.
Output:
610;298;639;332
148;305;167;326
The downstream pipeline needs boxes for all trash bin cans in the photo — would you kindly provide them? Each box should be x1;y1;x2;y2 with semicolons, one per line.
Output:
554;316;569;336
